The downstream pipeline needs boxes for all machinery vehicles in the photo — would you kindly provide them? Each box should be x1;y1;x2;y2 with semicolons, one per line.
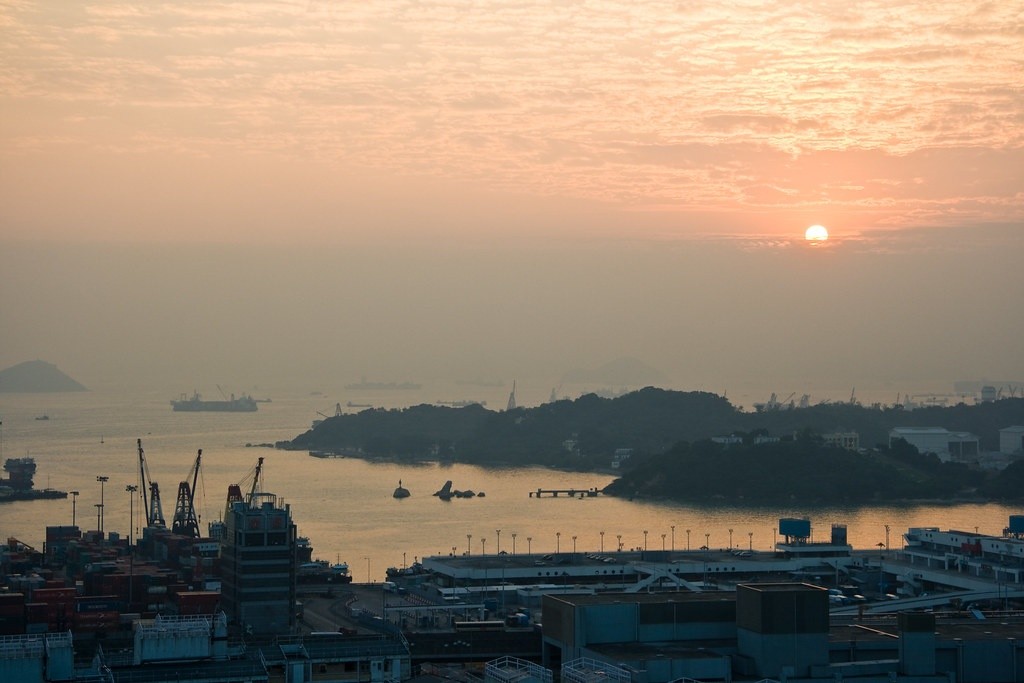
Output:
751;385;1018;414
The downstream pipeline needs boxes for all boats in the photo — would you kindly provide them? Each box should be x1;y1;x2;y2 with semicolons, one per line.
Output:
170;395;258;412
296;537;352;586
36;416;48;420
136;539;221;575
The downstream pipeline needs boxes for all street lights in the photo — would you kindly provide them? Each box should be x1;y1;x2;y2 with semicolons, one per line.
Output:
452;530;532;558
94;504;104;532
876;543;885;594
364;552;417;584
97;476;109;532
556;531;624;553
70;492;79;526
126;485;138;545
644;526;777;552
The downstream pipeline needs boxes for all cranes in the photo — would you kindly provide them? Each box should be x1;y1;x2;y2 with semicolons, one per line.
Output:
238;457;263;507
316;402;342;417
137;439;166;539
216;384;234;401
507;380;517;410
172;449;209;538
